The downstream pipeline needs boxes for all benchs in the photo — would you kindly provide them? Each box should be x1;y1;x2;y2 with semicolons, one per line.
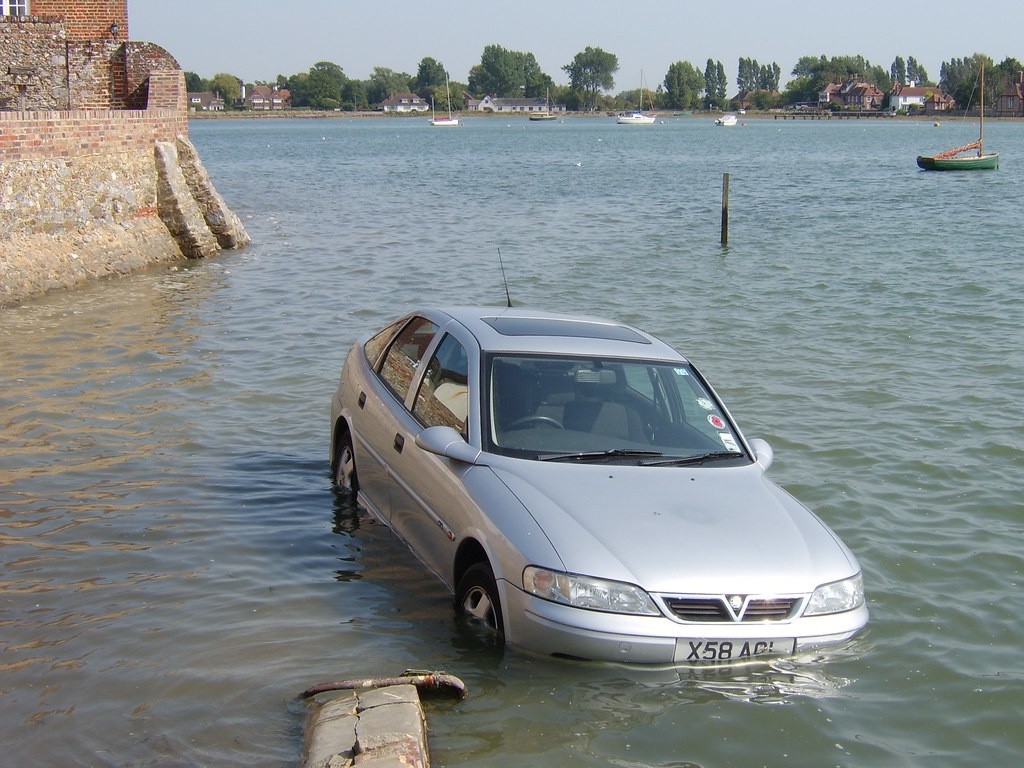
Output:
445;359;623;422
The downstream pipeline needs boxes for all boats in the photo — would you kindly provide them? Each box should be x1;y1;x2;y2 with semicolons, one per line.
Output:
715;114;738;126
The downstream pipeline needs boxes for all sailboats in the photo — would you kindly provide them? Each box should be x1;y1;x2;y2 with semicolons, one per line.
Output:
428;73;459;126
917;62;999;170
530;86;560;121
615;65;656;124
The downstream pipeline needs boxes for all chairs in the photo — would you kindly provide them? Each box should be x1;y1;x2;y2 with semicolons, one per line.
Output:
563;371;649;444
496;362;543;427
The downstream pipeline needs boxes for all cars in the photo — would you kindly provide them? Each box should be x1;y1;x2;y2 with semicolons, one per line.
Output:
327;305;870;671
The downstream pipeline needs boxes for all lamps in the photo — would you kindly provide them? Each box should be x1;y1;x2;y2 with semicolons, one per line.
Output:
110;20;118;34
87;39;94;54
